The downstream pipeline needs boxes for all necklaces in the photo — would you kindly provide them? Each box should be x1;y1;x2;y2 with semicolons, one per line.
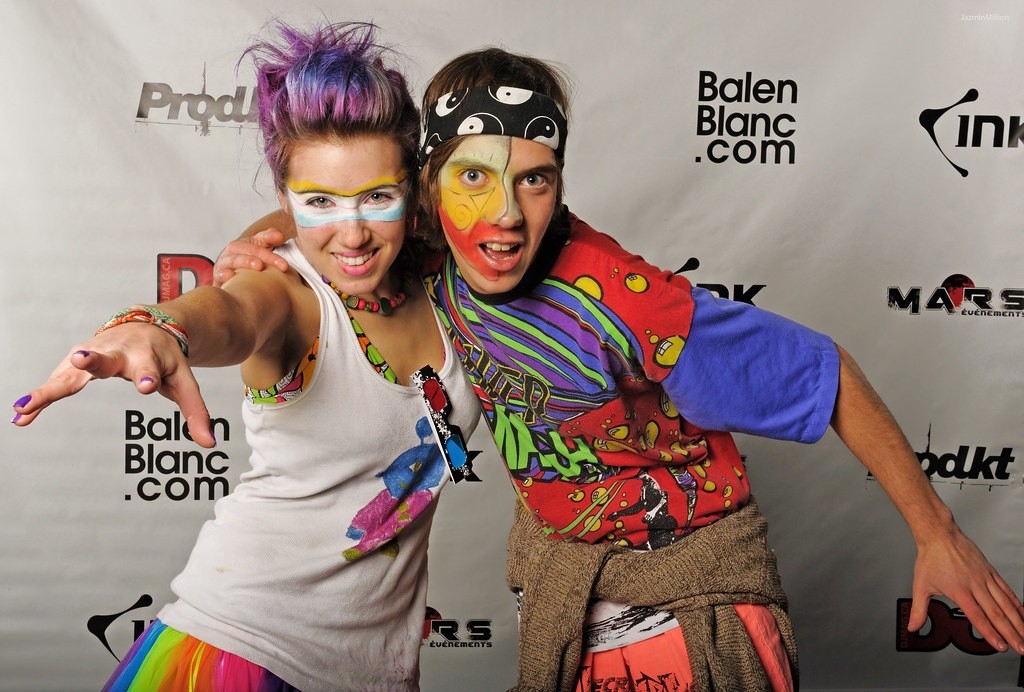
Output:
322;275;414;315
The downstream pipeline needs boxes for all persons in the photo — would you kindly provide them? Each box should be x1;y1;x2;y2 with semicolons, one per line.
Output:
11;18;481;692
212;45;1024;692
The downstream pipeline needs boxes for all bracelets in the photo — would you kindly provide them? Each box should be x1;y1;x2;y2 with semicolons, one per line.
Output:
94;305;189;356
